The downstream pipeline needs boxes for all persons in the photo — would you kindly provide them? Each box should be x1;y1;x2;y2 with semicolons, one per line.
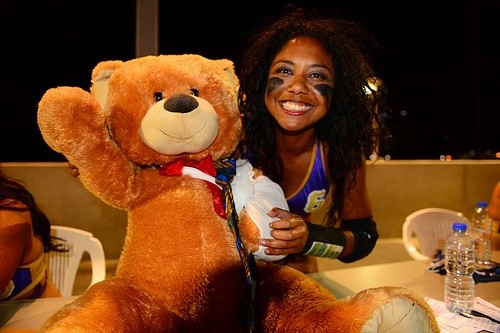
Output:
66;14;393;274
0;168;70;301
487;182;499;218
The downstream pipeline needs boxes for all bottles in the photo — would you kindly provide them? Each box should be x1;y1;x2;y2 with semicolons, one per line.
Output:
471;202;492;264
444;223;475;314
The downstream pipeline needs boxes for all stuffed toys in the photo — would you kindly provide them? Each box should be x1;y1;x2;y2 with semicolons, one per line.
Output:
38;50;441;333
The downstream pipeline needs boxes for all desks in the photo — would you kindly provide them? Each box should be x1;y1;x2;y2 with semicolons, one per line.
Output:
0;260;500;333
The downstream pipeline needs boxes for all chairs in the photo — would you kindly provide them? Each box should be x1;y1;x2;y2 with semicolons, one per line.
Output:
402;207;469;260
50;225;106;296
